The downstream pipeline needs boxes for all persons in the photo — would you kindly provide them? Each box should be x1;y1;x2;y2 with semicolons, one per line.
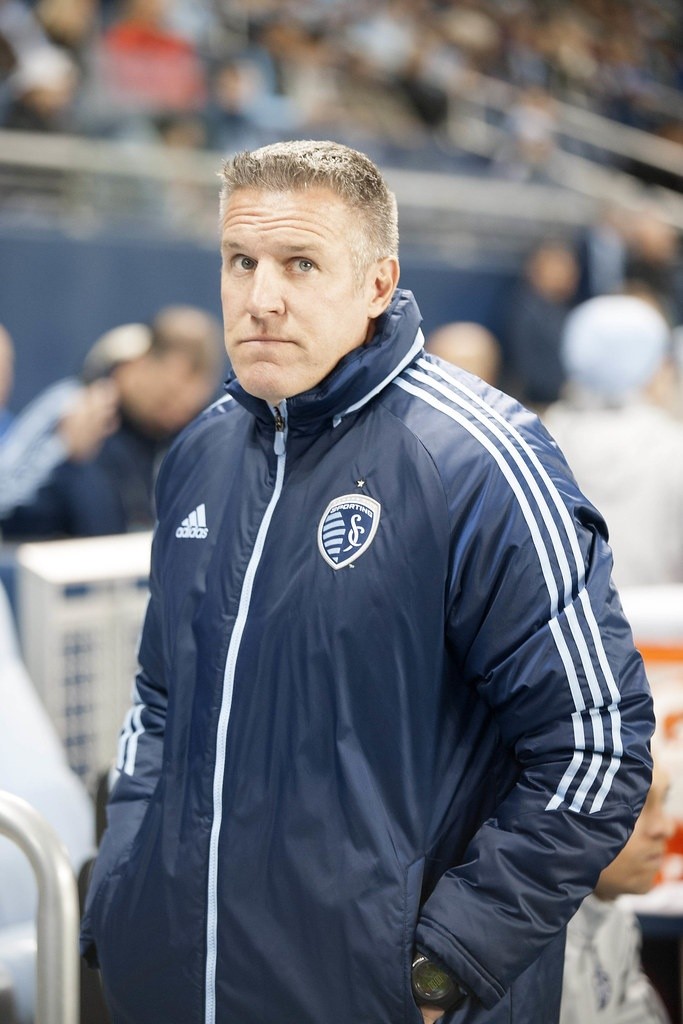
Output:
0;1;682;589
559;736;673;1024
82;140;655;1024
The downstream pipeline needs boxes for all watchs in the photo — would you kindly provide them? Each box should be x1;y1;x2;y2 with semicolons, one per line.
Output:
411;953;466;1011
